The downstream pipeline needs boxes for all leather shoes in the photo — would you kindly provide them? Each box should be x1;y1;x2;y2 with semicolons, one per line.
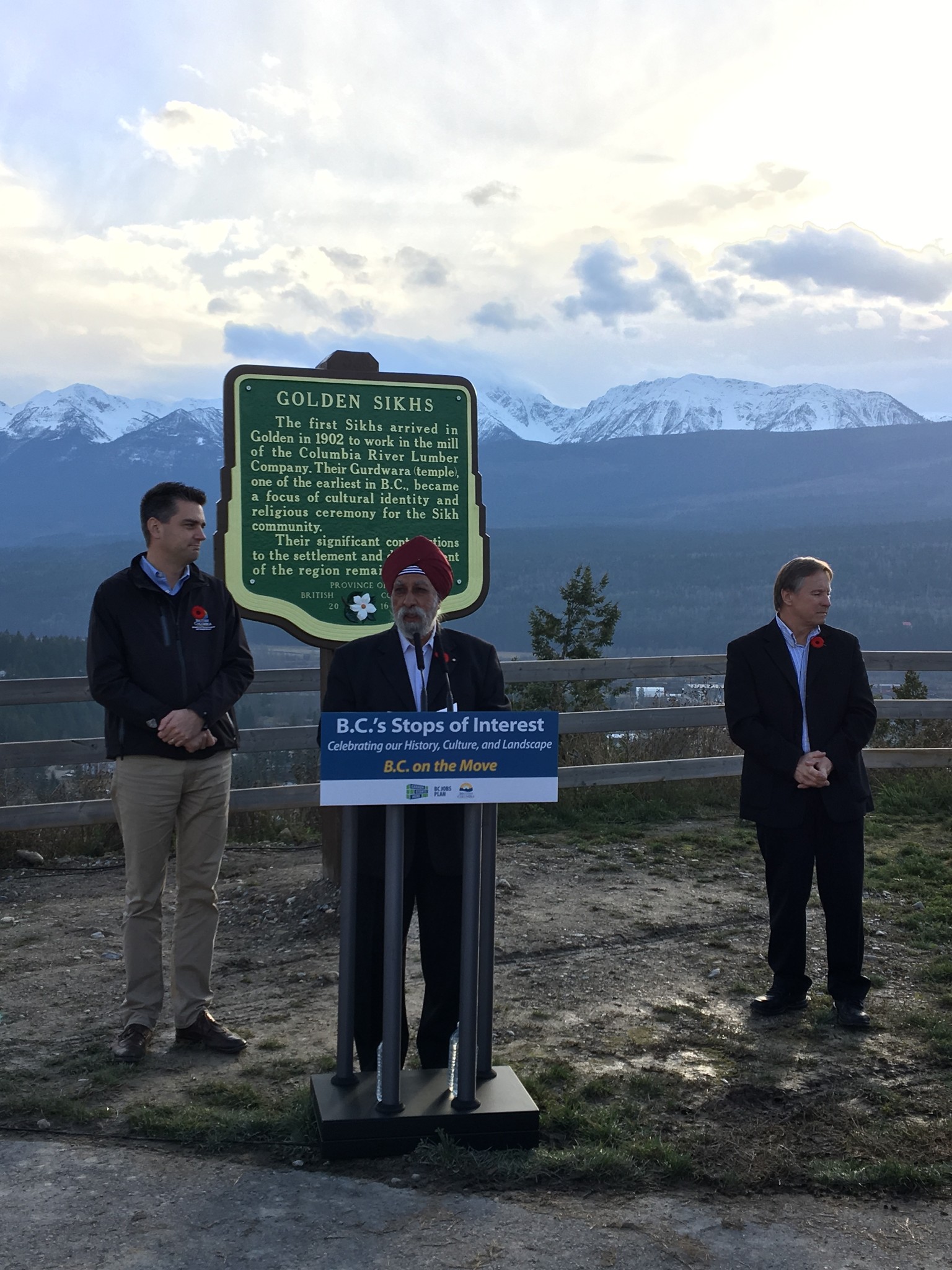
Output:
175;1011;249;1051
112;1024;151;1061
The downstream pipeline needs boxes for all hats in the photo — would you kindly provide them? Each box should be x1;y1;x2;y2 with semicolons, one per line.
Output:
382;536;454;602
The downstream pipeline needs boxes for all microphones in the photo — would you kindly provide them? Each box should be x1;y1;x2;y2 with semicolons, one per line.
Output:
433;634;454;712
413;632;429;711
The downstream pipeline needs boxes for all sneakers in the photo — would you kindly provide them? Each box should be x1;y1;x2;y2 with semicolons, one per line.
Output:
751;990;809;1013
833;997;872;1026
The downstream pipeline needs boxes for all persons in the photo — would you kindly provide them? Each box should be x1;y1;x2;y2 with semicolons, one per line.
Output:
314;535;511;1075
86;481;248;1065
723;556;878;1028
